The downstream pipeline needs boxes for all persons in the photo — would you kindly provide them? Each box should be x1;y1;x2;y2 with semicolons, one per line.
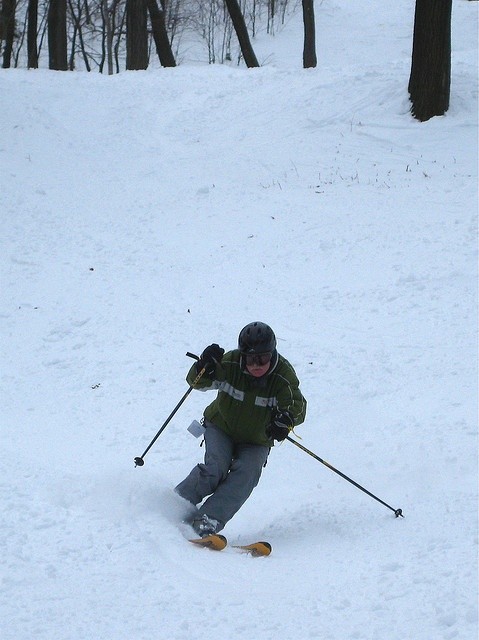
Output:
172;320;308;541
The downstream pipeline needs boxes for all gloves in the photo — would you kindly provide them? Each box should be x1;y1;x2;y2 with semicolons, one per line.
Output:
264;409;295;442
186;343;225;380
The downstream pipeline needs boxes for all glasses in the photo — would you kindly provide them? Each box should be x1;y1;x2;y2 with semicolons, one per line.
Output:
240;348;274;366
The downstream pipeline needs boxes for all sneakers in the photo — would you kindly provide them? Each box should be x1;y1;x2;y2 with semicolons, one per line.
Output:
183;510;220;537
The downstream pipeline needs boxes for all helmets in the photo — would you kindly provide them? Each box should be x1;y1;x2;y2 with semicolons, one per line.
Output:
238;322;277;354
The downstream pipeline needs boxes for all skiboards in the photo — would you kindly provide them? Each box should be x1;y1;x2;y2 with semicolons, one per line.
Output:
181;526;271;557
